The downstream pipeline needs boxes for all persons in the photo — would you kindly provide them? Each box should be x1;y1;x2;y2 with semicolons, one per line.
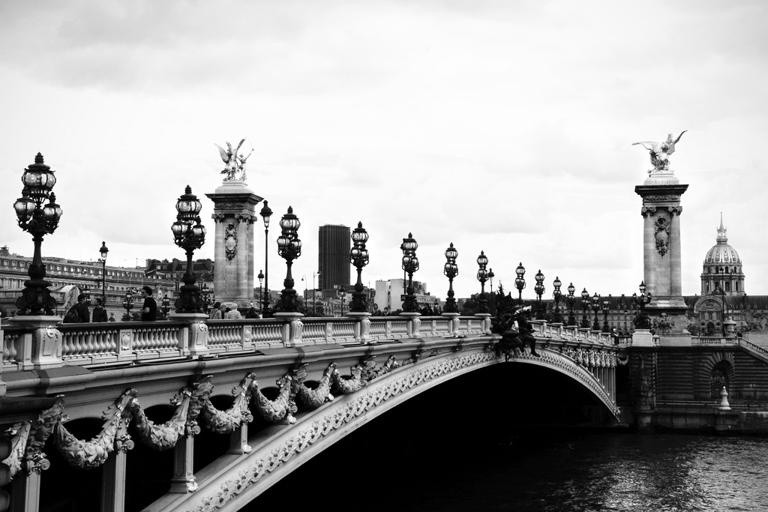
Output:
69;294;107;323
371;304;381;316
137;286;157;321
210;302;260;319
494;317;541;357
109;313;116;321
383;307;390;316
422;303;432;316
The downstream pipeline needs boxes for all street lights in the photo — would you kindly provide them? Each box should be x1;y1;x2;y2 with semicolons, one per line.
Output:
400;232;652;332
171;184;207;313
99;241;110;307
13;151;63;316
276;205;302;312
348;221;369;311
257;200;273;313
338;285;346;316
